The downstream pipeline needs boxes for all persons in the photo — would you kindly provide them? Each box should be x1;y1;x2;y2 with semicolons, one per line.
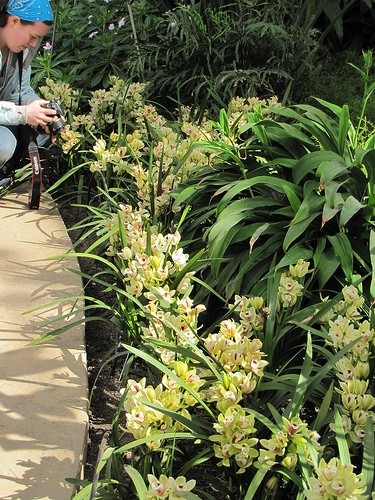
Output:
0;0;66;172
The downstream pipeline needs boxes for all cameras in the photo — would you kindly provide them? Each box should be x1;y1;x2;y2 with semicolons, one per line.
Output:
35;99;66;136
